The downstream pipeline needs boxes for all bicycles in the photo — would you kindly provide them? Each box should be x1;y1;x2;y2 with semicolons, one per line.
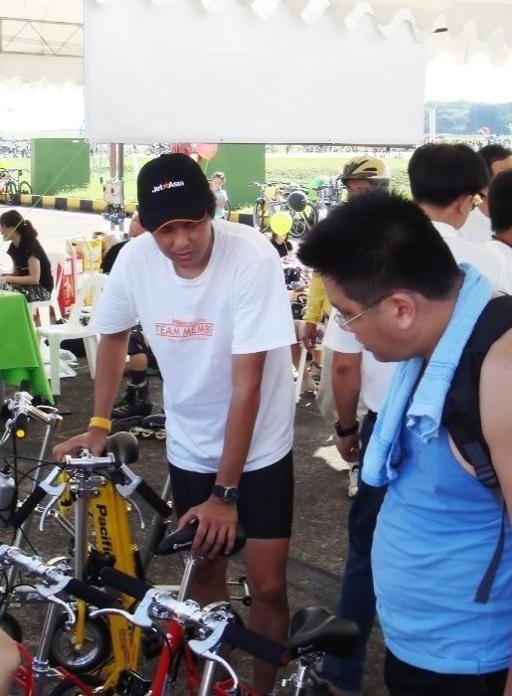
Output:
0;167;33;206
249;175;349;239
208;179;232;220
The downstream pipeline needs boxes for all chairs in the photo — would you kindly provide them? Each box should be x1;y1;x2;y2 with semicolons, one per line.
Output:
23;253;64;326
35;269;107;396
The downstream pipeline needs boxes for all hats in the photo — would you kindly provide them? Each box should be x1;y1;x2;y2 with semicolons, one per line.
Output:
138;152;210;234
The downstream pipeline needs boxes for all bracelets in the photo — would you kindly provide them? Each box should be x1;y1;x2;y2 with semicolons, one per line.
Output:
88;416;112;434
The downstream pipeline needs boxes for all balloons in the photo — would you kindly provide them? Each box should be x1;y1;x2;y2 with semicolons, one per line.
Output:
287;189;307;213
195;143;218;159
270;211;293;235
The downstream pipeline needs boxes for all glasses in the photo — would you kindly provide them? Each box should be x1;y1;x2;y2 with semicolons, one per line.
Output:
333;291;396;333
467;189;486;208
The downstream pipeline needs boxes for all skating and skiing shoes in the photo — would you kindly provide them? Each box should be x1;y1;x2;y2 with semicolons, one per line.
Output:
129;409;165;440
299;377;316;400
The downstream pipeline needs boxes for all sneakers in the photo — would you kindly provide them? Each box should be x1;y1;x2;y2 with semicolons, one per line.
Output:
309;361;322;380
111;378;153;419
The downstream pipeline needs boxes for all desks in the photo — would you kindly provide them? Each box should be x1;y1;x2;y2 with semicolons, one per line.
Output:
0;286;54;405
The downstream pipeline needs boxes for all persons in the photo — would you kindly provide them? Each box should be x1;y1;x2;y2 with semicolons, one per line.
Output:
52;152;297;696
0;209;54;303
301;154;390;498
209;172;228;220
127;205;144;238
321;143;512;696
458;143;512;245
267;231;318;395
487;167;512;254
295;186;512;696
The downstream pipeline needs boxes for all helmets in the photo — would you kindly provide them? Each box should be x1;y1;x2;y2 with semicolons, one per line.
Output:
335;154;391;181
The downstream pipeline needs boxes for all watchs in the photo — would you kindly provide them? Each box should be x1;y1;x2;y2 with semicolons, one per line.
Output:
212;485;240;505
334;420;359;438
